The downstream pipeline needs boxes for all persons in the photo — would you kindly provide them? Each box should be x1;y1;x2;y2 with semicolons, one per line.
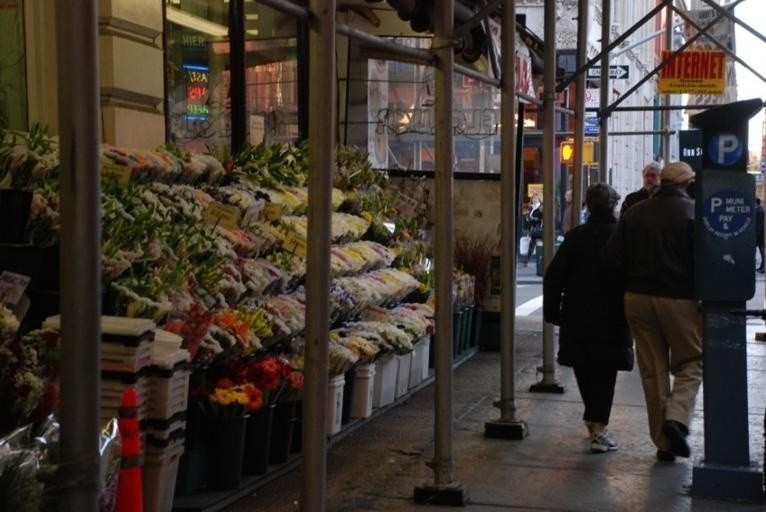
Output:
609;162;703;463
754;197;766;274
521;195;543;265
619;163;662;219
562;187;574;235
542;183;634;451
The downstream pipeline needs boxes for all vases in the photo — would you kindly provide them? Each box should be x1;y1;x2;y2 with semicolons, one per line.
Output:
409;346;421;389
275;400;296;464
420;340;429;382
245;405;273;477
371;359;398;409
393;353;411;399
349;363;377;419
326;374;345;437
342;369;357;425
206;416;246;491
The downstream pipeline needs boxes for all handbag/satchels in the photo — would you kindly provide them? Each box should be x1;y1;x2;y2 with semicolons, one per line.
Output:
555;281;569;324
530;218;544;241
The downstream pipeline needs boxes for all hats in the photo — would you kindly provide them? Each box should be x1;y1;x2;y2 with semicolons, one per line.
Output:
590;429;620;451
659;162;696;187
656;448;675;462
661;418;693;458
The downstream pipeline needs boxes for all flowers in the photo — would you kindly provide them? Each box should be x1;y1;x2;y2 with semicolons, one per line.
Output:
237;356;290;406
291;336;358;377
339;341;361;372
402;307;436;337
390;313;423;344
357;321;414;356
331;332;392;367
191;377;263;417
279;354;306;401
